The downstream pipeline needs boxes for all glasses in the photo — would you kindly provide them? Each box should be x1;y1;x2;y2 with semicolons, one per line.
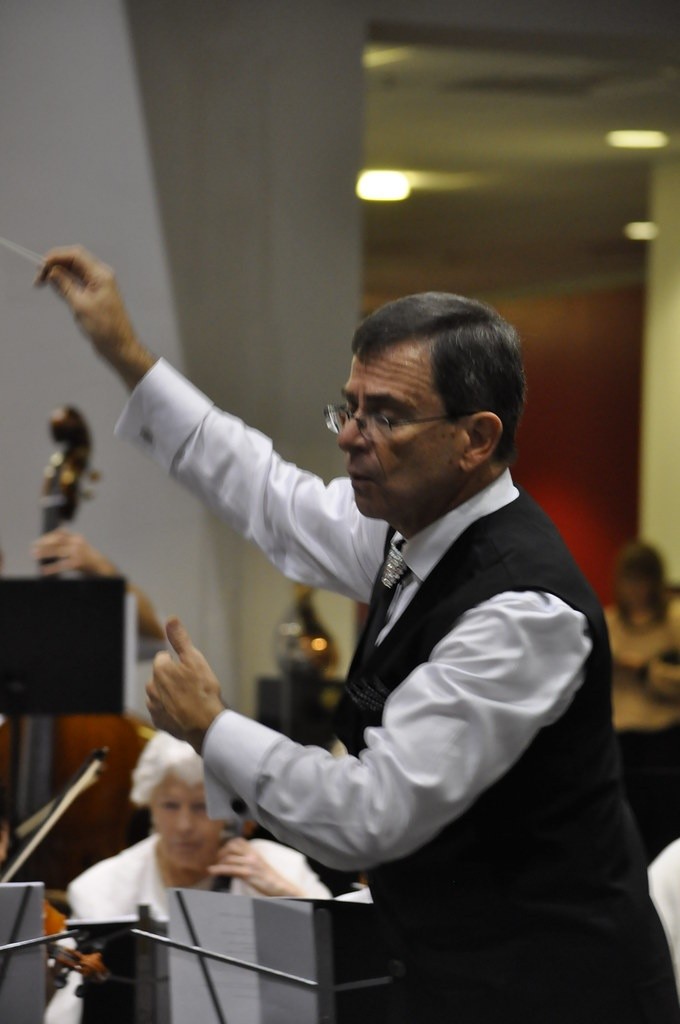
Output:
323;403;478;441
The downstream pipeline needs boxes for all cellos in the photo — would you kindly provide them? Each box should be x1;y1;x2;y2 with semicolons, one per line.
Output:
0;405;151;890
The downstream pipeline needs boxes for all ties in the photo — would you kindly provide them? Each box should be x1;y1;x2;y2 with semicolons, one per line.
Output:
349;539;412;684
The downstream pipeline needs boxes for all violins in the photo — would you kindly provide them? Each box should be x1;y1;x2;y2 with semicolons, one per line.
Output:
46;896;113;1000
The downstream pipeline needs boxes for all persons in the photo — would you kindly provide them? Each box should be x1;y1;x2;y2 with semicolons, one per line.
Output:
45;729;332;1024
0;786;67;947
598;544;680;865
35;529;164;669
29;245;680;1023
